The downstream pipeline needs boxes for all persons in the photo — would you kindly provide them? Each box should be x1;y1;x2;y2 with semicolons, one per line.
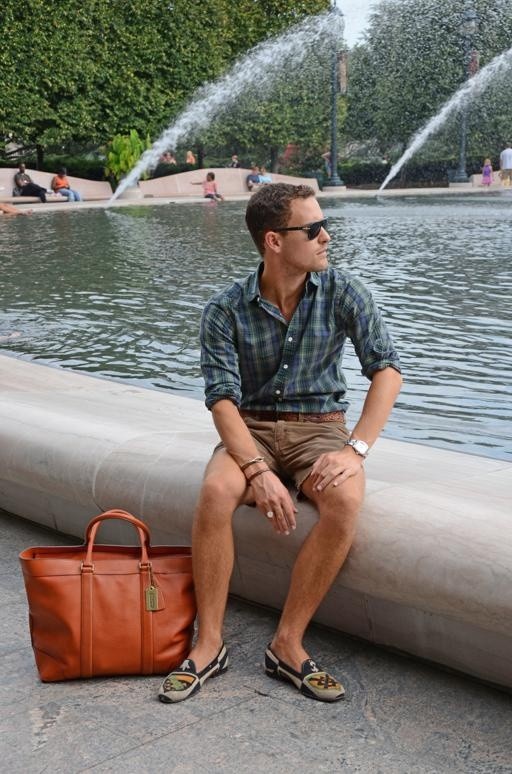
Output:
481;158;495;185
185;149;196;165
260;164;273;185
156;180;406;704
161;150;179;166
224;154;241;167
498;141;512;188
14;162;54;202
320;148;343;178
247;165;261;192
190;172;225;202
52;166;81;202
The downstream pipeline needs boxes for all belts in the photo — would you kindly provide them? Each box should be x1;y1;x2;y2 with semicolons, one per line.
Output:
239;409;343;423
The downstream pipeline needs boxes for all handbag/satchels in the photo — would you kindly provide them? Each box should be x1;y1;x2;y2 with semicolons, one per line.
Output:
17;507;198;683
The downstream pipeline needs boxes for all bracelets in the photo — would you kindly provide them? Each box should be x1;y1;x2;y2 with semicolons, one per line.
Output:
238;455;268;472
247;466;271;483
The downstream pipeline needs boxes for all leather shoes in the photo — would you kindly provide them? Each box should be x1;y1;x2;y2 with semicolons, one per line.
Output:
158;642;231;704
264;640;346;703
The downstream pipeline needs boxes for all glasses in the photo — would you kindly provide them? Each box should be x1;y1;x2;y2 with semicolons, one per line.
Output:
267;217;329;242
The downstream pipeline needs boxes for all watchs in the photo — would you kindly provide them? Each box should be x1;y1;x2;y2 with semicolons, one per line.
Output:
343;437;370;460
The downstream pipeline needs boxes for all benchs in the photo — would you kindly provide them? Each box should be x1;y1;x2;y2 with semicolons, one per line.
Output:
0;167;115;203
137;168;322;199
470;171;512;189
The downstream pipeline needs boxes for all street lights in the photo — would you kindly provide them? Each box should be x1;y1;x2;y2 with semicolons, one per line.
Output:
327;6;346;185
451;0;480;182
